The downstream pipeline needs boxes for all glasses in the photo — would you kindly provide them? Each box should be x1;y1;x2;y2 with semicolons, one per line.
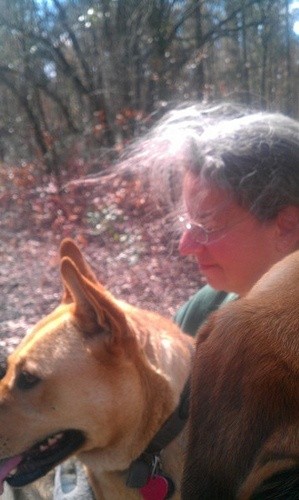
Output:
179;212;252;242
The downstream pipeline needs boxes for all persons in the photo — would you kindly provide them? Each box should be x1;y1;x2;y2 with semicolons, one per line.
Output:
168;111;299;339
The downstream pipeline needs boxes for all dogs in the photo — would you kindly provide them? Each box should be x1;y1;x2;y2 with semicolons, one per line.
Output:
0;236;299;500
181;249;299;500
